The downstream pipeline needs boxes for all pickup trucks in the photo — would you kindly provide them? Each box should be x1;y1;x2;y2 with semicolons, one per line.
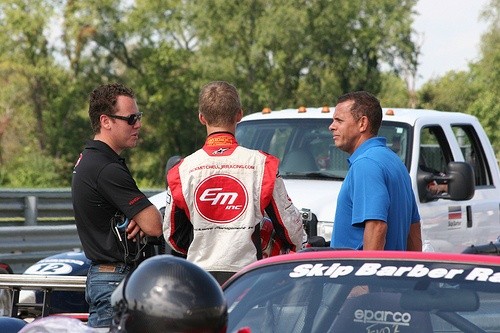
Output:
145;105;498;257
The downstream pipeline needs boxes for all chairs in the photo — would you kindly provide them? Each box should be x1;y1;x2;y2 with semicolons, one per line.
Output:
282;148;321;171
329;292;433;333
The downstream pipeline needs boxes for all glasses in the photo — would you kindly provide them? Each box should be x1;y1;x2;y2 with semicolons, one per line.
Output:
106;112;143;126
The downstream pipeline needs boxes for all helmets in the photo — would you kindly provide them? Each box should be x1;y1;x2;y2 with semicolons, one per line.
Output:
111;254;228;333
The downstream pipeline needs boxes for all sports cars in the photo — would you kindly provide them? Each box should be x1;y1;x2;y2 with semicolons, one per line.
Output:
211;250;500;333
0;251;110;333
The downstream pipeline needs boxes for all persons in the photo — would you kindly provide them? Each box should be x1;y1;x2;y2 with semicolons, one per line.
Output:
163;80;310;286
71;83;163;328
108;253;228;333
329;91;422;251
419;164;448;194
